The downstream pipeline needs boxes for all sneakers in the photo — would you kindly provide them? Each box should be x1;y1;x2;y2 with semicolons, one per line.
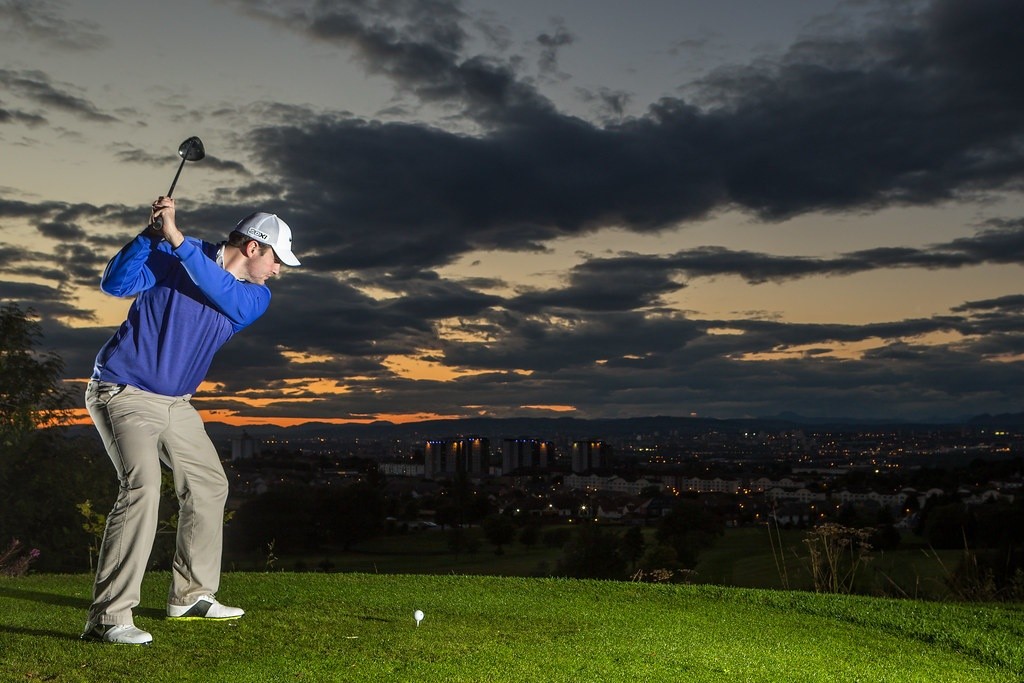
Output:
166;593;245;622
81;620;153;646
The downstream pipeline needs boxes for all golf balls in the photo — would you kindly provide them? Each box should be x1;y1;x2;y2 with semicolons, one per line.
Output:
412;609;425;622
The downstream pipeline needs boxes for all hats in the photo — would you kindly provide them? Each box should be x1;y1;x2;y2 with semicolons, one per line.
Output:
234;211;302;266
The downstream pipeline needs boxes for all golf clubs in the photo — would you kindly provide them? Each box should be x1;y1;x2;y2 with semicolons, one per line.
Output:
152;134;207;230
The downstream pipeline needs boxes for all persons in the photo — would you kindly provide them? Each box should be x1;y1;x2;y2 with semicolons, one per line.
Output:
85;196;301;644
